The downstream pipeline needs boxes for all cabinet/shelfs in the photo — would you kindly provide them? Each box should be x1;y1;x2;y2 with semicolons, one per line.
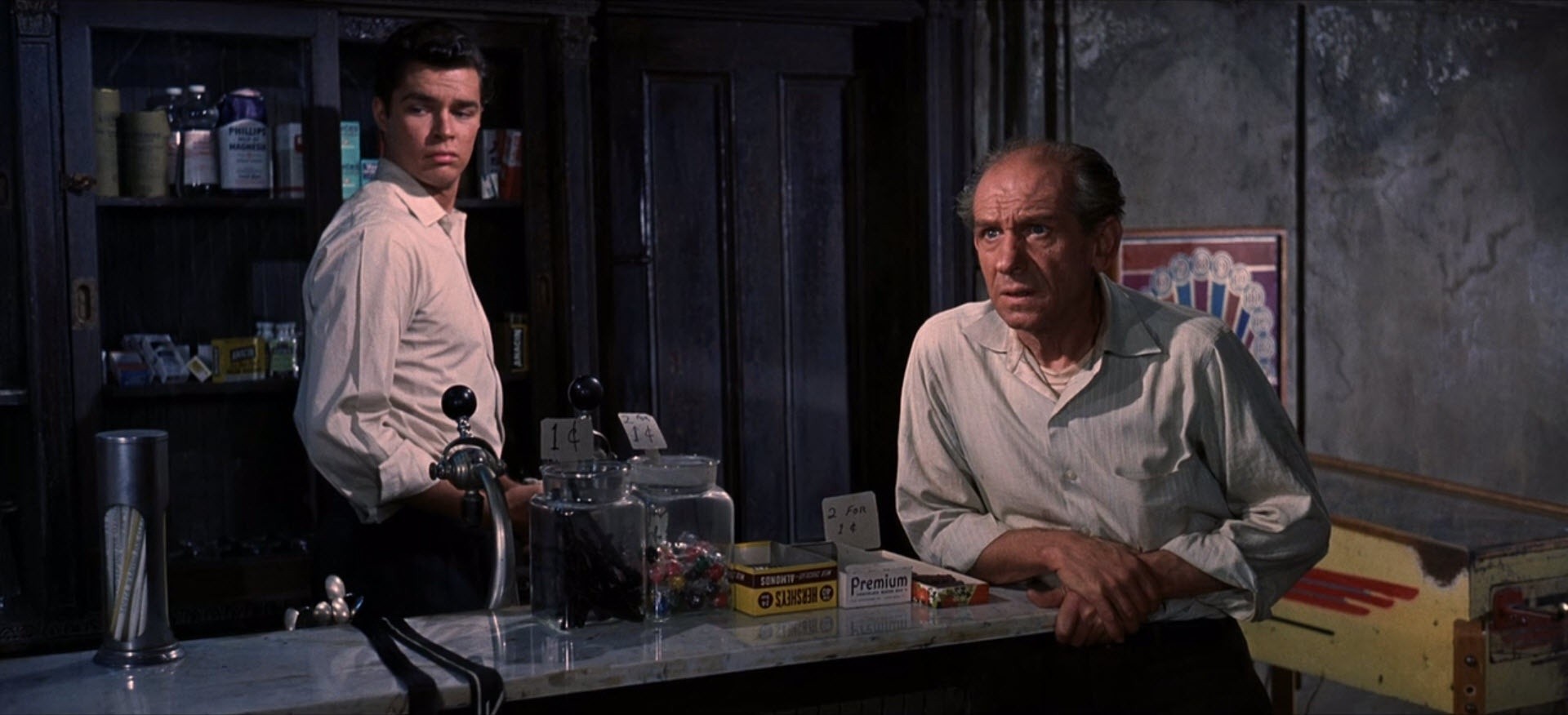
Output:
0;0;979;645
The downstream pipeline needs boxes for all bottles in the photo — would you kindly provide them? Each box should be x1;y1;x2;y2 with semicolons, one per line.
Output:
630;452;736;623
527;459;648;637
93;86;221;199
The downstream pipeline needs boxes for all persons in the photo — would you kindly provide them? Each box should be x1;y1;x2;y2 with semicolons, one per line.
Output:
891;138;1333;715
293;30;542;614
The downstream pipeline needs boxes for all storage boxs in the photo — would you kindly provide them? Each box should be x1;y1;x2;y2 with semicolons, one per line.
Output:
497;129;524;199
868;549;995;608
792;539;915;611
477;128;499;199
276;123;306;200
141;334;190;384
723;540;838;618
108;351;151;390
132;334;160;386
207;337;267;384
